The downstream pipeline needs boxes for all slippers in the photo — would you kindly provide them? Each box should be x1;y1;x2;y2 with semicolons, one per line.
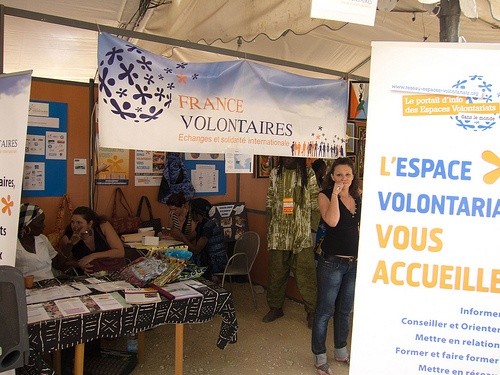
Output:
336;357;349;365
314;363;333;375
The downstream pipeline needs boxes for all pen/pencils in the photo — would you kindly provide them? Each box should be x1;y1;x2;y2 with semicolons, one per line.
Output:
69;284;80;291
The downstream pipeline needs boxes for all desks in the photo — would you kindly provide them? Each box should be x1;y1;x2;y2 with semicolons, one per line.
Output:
23;270;240;375
117;233;189;261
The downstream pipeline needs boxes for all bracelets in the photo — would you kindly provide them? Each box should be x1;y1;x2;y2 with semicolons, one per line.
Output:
332;193;337;196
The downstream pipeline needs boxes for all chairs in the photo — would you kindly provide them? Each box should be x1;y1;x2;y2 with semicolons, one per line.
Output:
212;230;261;313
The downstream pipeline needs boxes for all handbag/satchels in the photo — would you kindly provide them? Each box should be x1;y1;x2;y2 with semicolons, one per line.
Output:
47;194;72;247
138;196;161;237
157;159;196;203
108;187;143;234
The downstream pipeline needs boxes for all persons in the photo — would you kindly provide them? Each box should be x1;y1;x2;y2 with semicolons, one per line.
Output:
158;193;228;278
60;206;140;276
262;156;322;329
15;200;93;289
311;156;361;375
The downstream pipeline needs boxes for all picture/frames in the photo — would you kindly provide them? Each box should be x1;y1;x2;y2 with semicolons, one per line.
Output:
347;155;358;165
346;121;356;156
255;155;274;180
358;125;367;179
348;80;370;121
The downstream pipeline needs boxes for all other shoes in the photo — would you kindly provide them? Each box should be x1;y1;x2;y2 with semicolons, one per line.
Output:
263;308;283;322
306;312;314;328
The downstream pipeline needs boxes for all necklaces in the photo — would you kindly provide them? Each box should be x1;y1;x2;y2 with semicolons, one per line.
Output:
179;209;187;222
